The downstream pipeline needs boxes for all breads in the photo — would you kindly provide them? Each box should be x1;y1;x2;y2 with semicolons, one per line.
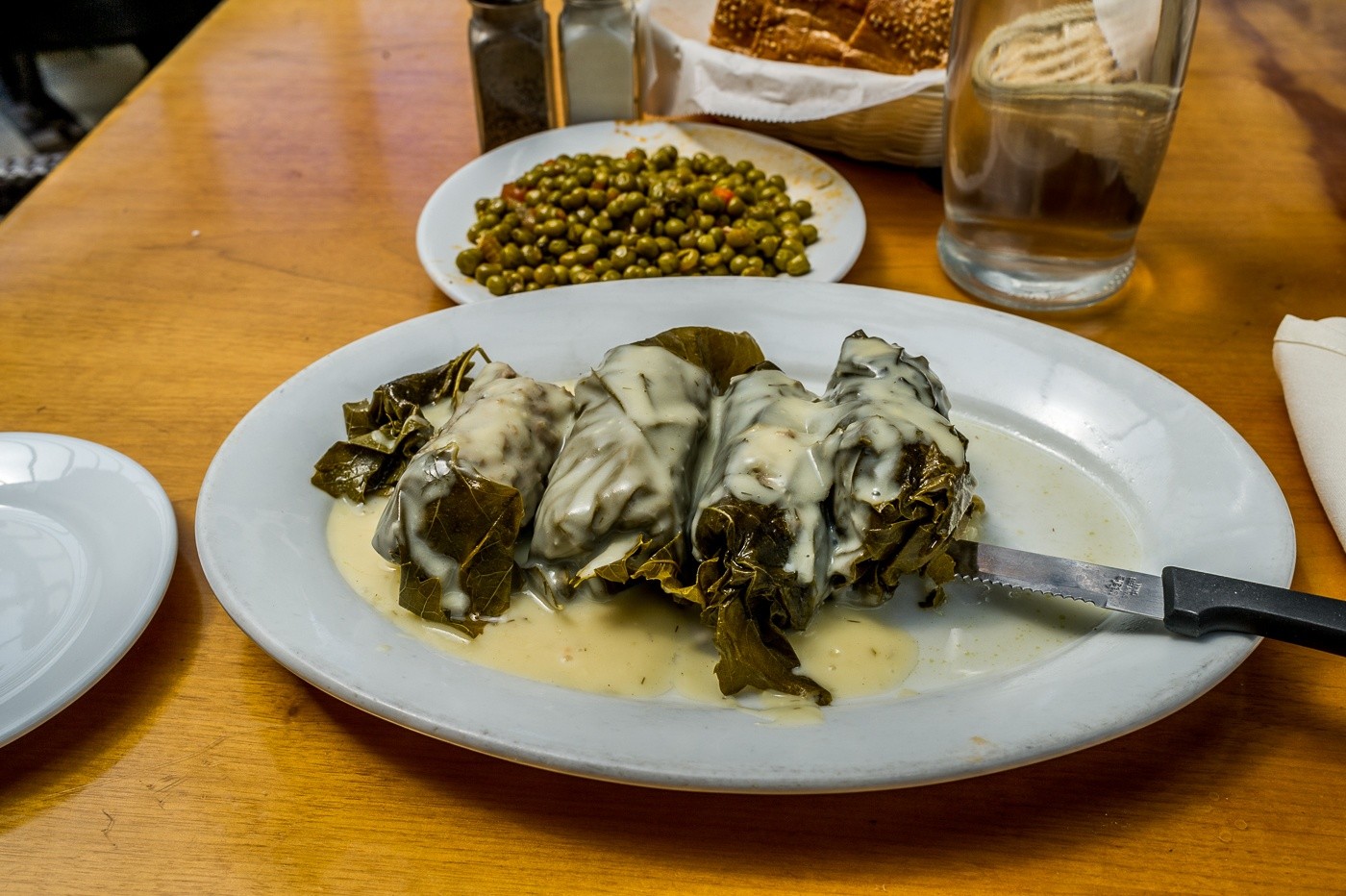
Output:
707;0;966;78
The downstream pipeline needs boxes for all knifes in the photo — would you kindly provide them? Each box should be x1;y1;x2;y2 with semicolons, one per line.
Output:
946;542;1345;660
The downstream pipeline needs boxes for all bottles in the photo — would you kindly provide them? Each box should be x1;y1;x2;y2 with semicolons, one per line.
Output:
467;0;557;155
557;0;641;127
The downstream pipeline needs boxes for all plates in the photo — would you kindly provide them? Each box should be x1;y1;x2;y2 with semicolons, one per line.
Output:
194;277;1297;795
0;428;178;748
415;119;867;307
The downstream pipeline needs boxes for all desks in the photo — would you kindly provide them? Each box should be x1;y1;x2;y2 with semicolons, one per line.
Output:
0;0;1345;896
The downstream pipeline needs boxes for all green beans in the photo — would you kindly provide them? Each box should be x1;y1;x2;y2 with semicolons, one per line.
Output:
456;146;818;293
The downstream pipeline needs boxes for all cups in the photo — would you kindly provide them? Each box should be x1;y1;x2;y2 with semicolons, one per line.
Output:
934;0;1203;311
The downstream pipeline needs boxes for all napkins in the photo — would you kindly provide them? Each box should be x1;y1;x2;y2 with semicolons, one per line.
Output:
1272;314;1346;548
